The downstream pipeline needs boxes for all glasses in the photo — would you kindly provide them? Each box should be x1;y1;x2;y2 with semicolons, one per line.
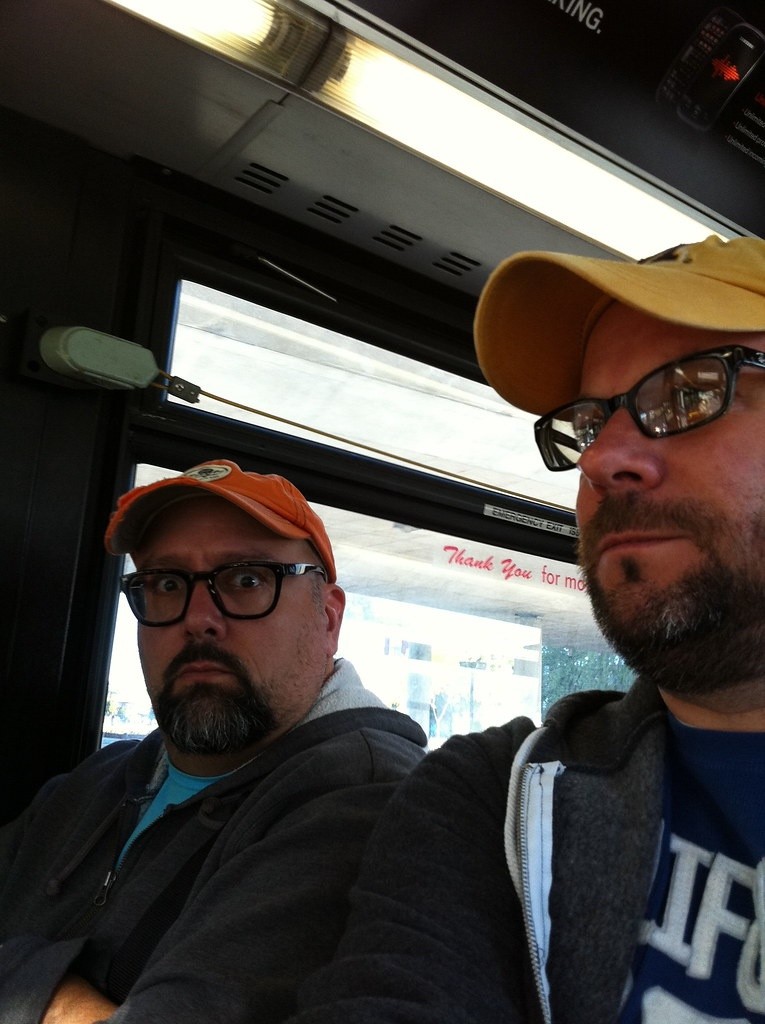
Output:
534;344;765;471
120;563;329;628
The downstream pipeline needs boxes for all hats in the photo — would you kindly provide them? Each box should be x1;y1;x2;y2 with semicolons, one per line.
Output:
103;458;338;583
472;234;765;418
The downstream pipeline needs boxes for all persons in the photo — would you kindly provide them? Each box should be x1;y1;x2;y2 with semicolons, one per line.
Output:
301;237;765;1024
0;458;427;1023
